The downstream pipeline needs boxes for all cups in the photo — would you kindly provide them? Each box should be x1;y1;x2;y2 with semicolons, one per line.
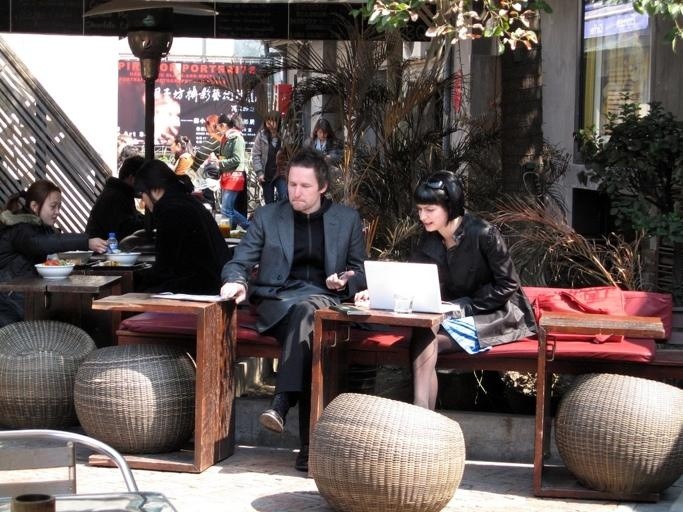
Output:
8;494;55;512
391;287;414;314
218;217;230;237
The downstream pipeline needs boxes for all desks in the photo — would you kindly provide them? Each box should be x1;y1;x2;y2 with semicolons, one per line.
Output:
88;290;238;473
307;301;447;478
534;311;668;503
0;492;177;512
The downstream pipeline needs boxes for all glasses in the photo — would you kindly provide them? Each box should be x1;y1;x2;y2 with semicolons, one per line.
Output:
418;178;447;190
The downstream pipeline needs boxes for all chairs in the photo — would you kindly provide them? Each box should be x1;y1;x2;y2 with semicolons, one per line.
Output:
1;428;137;498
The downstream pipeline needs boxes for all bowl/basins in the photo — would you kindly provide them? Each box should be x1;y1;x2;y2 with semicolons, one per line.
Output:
35;263;74;281
59;249;94;265
103;251;140;266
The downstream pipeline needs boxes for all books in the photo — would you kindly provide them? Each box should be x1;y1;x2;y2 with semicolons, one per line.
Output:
329;305;370;316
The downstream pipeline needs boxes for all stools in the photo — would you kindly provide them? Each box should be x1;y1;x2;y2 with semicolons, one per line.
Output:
309;391;466;512
0;319;98;429
73;343;196;455
553;372;683;497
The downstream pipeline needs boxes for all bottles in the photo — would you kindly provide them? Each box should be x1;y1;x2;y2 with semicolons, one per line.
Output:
106;233;117;251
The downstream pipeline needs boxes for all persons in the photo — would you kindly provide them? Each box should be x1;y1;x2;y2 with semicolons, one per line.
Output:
169;109;344;232
121;159;233;320
0;180;113;346
85;156;146;242
409;169;540;411
220;150;368;472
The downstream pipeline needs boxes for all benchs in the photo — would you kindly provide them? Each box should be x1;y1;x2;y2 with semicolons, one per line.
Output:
117;312;682;408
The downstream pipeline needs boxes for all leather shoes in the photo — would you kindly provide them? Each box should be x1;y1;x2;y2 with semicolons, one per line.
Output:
295;445;308;470
257;409;285;433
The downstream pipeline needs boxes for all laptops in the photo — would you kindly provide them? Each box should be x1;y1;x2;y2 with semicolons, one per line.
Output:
364;261;461;314
